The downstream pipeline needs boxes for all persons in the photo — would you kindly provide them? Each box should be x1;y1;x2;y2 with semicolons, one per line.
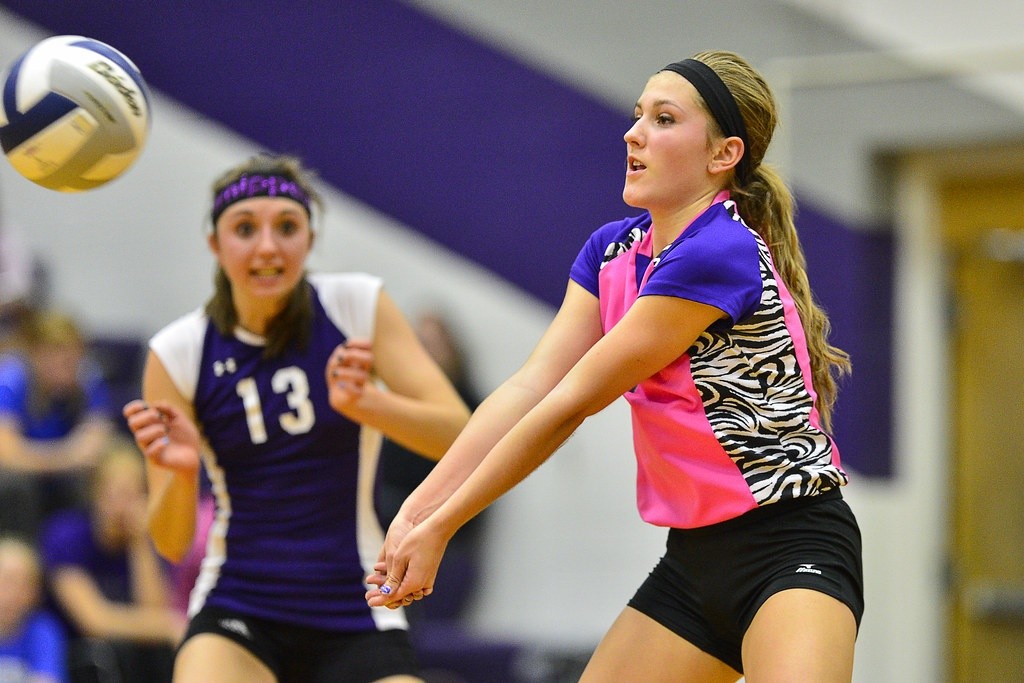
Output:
122;156;471;683
371;314;490;683
365;51;865;683
0;301;216;683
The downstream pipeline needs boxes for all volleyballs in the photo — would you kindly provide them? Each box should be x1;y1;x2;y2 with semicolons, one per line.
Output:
1;32;154;197
